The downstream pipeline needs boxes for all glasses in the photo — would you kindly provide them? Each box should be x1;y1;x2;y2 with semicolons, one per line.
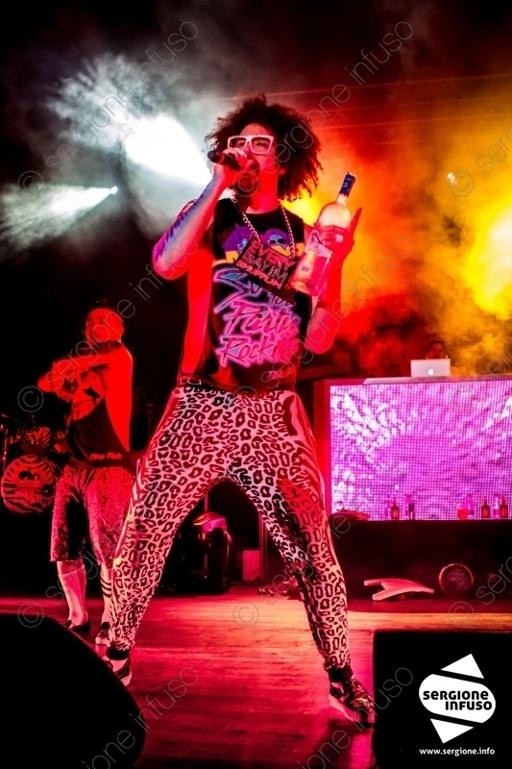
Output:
226;134;276;156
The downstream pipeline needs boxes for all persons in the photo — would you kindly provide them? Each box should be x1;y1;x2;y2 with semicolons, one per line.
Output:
101;95;376;725
36;306;134;643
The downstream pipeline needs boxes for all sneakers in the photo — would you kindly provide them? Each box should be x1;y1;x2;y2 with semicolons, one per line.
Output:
95;622;132;687
63;619;91;635
328;673;375;731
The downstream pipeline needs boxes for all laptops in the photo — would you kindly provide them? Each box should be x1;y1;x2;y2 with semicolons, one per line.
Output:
410;357;451;378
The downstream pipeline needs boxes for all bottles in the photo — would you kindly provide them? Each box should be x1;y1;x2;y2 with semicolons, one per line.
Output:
288;174;356;296
390;493;508;520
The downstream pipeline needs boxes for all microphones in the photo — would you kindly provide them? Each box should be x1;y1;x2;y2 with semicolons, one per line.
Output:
206;147;239;172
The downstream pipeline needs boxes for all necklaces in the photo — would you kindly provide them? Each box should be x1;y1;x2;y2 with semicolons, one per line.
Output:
230;195;296;260
